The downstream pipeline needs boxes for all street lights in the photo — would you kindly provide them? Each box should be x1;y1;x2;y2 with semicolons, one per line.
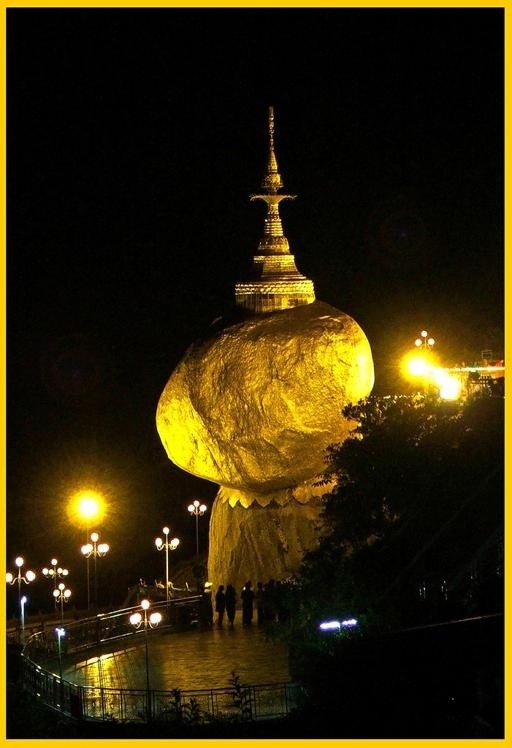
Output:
154;526;181;600
52;582;72;626
187;499;209;592
42;557;68;618
129;599;163;713
414;330;436;395
55;627;68;704
6;555;35;632
80;532;109;612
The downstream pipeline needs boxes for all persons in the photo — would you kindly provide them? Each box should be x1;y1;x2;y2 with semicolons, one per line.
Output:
215;578;290;628
